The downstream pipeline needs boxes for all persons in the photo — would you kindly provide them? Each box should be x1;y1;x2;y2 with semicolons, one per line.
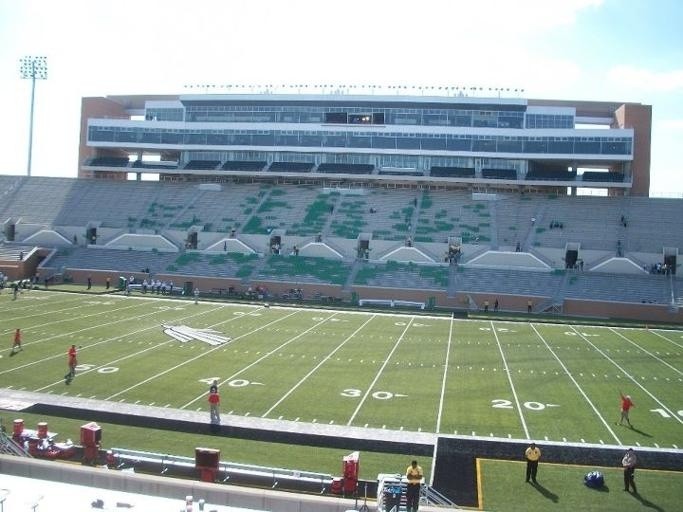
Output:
493;297;499;312
484;300;489;312
525;442;540;482
12;329;24;354
405;460;422;512
642;258;673;276
64;345;78;381
209;380;217;392
619;390;635;426
621;448;636;492
527;299;533;313
0;271;37;299
208;389;220;421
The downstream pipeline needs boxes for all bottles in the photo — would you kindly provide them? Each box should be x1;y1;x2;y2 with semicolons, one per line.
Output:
198;498;205;511
185;495;193;512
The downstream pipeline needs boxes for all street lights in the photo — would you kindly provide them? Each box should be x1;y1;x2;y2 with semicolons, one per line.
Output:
20;56;47;176
